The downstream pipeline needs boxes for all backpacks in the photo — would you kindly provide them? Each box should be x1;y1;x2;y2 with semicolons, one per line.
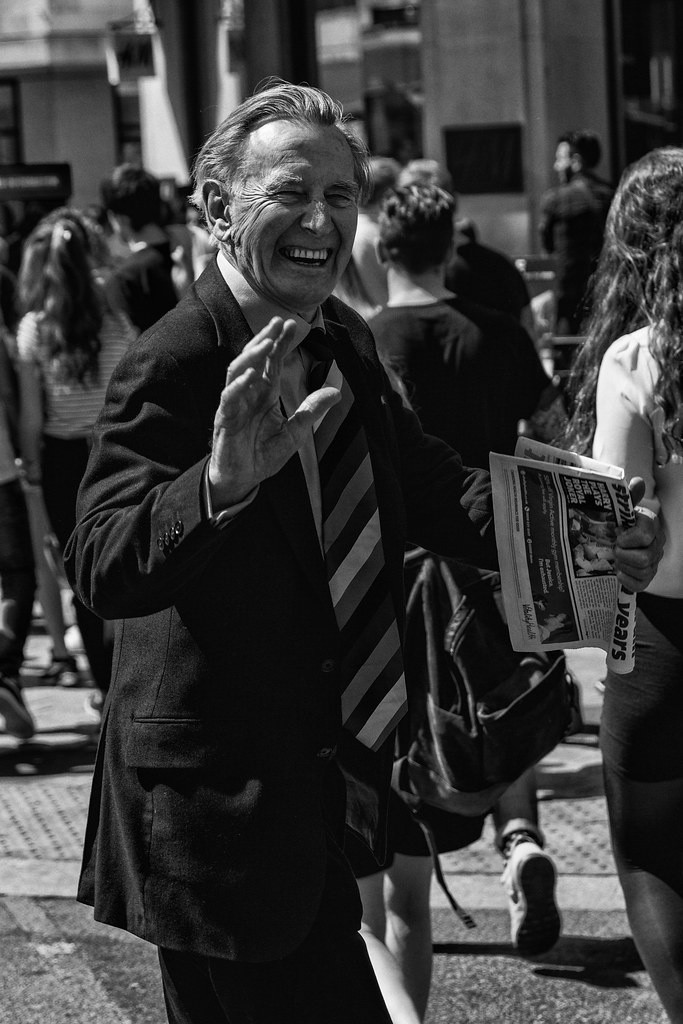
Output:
398;534;582;814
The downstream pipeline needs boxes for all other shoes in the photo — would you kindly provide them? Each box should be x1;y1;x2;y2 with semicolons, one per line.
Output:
497;841;561;955
89;690;106;713
0;676;39;741
38;655;81;687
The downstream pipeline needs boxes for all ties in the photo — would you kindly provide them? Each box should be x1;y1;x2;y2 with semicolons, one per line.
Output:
297;328;410;754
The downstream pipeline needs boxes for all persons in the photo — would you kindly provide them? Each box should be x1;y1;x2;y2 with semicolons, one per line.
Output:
537;132;615;369
344;548;484;1024
567;148;683;1024
62;83;668;1024
0;163;180;739
330;159;570;958
